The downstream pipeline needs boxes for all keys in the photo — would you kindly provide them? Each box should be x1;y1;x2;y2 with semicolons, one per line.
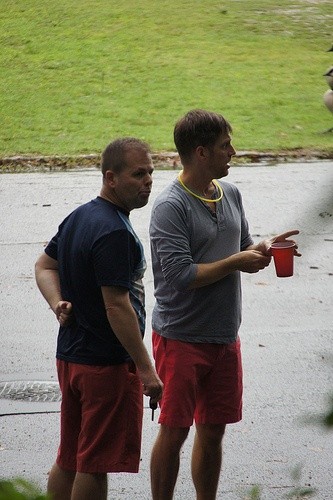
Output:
149;397;158;421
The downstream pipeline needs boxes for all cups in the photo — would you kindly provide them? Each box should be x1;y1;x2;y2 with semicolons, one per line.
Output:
270;242;296;277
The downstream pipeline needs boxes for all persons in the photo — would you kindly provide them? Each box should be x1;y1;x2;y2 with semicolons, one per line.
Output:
149;109;302;500
34;137;164;500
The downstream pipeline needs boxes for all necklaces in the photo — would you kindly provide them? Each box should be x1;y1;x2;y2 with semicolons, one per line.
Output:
178;170;223;202
